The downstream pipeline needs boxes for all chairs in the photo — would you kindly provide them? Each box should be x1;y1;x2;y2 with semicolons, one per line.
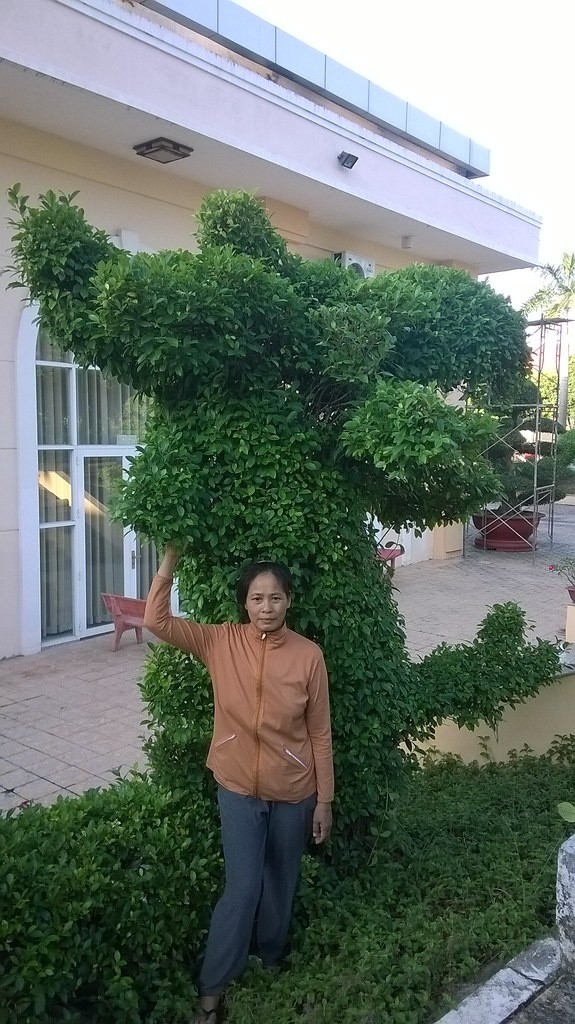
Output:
101;593;147;651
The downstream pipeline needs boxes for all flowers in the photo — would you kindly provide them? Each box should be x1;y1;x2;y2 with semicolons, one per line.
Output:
549;558;575;586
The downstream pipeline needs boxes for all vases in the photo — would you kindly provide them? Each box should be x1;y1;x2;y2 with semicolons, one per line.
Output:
566;586;575;604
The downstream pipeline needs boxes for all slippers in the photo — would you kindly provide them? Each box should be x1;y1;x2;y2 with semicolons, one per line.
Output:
197;995;222;1024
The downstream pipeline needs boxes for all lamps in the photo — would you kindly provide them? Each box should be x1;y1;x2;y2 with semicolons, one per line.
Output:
133;136;193;165
338;151;358;169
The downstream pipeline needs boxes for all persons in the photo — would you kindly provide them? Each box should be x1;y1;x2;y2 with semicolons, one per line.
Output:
141;544;335;1023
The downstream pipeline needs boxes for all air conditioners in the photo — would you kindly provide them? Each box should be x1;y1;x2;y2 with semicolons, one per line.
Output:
334;250;375;280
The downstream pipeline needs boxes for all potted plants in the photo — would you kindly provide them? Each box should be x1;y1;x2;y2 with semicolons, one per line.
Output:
469;374;575;550
